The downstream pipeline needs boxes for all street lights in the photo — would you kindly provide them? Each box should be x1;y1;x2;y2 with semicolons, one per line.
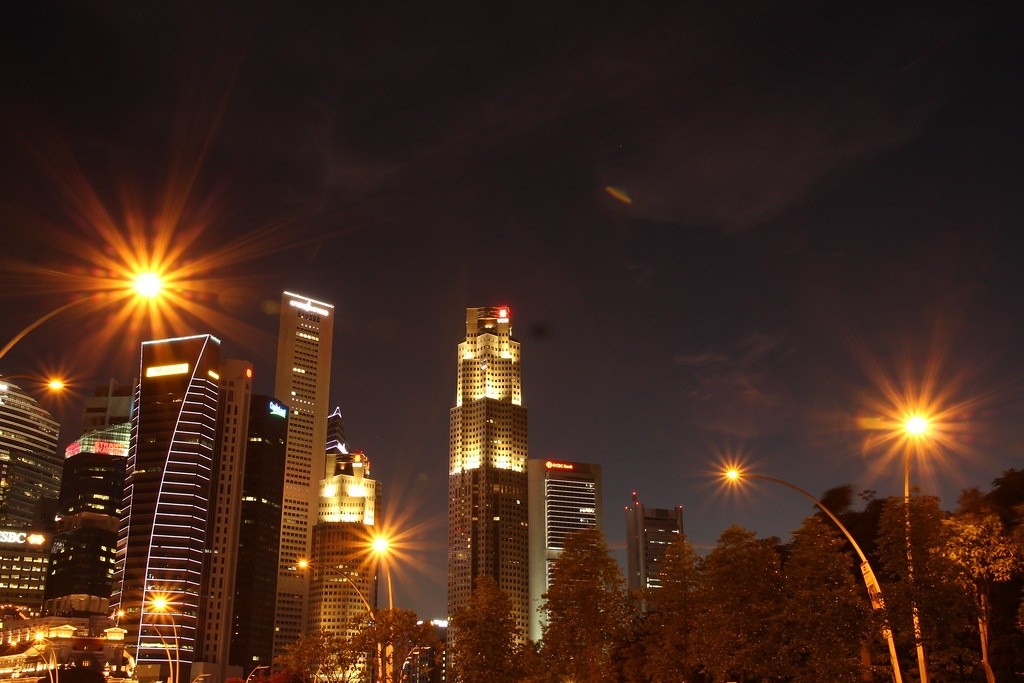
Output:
11;641;54;683
153;596;179;683
724;467;902;683
297;559;383;683
118;610;174;683
371;535;396;683
894;407;937;683
36;633;58;683
